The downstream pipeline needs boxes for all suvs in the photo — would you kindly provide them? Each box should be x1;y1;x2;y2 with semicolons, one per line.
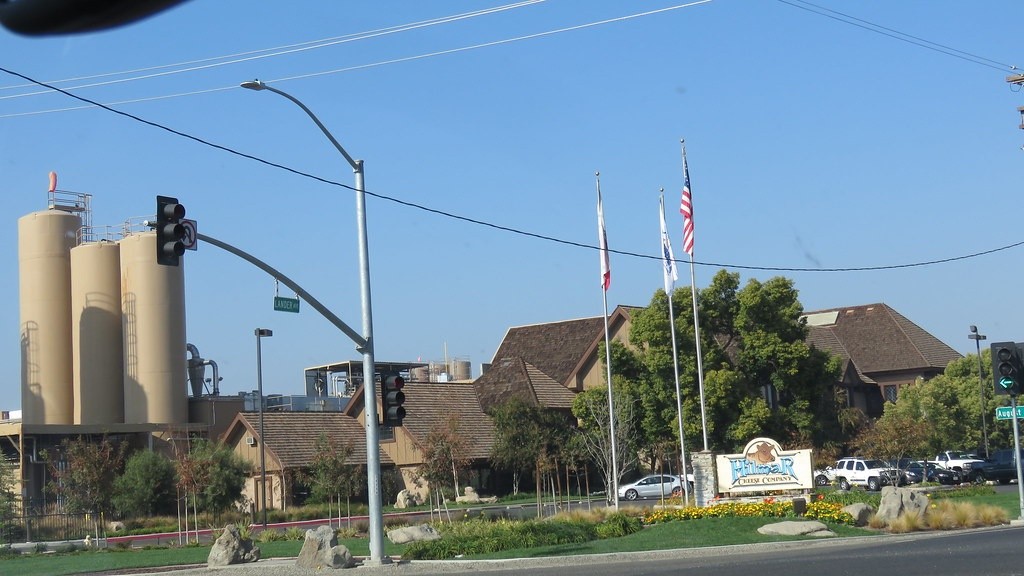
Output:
832;455;905;491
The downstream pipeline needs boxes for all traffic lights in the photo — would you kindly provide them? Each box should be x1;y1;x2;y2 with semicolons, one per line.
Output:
990;341;1022;395
380;364;406;427
156;195;186;266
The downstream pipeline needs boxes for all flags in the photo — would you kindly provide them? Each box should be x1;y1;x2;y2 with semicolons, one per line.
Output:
660;203;678;298
680;159;694;256
597;194;610;291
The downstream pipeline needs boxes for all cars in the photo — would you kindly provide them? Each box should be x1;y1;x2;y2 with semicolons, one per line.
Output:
897;445;1024;486
812;456;838;487
618;473;692;501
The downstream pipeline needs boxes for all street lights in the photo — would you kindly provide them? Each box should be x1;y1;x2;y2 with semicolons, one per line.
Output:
967;324;990;458
239;79;392;567
254;326;273;530
